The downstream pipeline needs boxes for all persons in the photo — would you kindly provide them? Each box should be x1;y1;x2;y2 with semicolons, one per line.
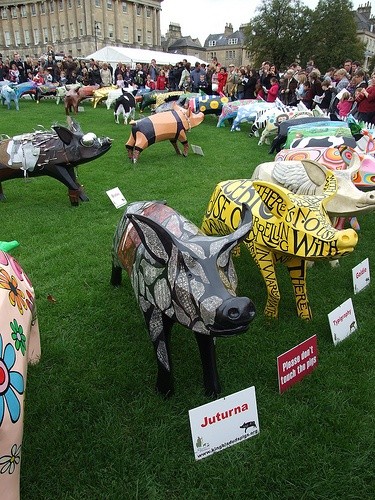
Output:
1;48;375;129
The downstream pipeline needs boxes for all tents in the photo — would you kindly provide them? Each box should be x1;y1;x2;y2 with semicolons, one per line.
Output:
86;45;210;84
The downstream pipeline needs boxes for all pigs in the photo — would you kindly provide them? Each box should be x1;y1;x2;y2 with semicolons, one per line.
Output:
0;80;375;500
